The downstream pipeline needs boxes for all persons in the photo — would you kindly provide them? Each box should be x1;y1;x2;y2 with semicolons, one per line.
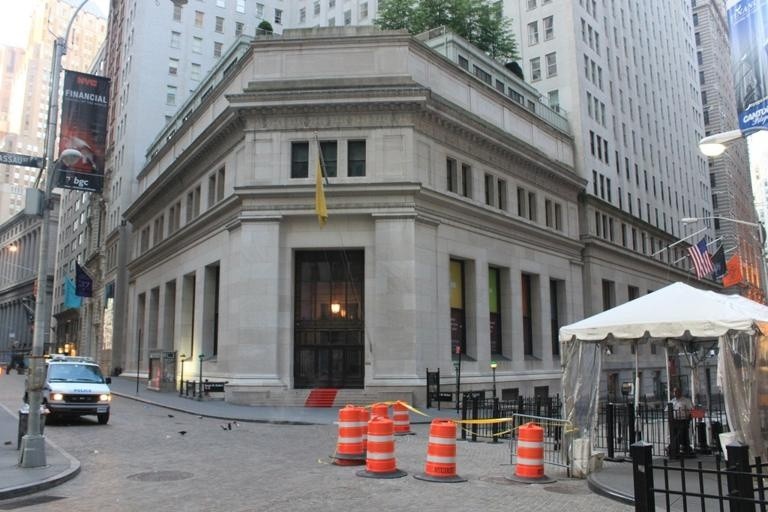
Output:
6;340;25;375
664;387;696;461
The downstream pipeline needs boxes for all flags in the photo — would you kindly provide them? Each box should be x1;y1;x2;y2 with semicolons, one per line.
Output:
74;262;94;299
63;276;83;308
722;251;743;288
314;150;328;229
25;306;35;325
688;235;715;281
710;244;728;281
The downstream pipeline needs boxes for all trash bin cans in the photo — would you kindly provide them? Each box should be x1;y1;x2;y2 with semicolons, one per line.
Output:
18;404;50;447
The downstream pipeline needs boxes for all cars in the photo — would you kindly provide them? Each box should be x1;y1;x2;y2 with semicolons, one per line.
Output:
25;354;112;425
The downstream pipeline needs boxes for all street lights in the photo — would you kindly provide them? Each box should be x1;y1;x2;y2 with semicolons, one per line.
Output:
18;144;84;467
681;216;768;289
698;126;768;157
